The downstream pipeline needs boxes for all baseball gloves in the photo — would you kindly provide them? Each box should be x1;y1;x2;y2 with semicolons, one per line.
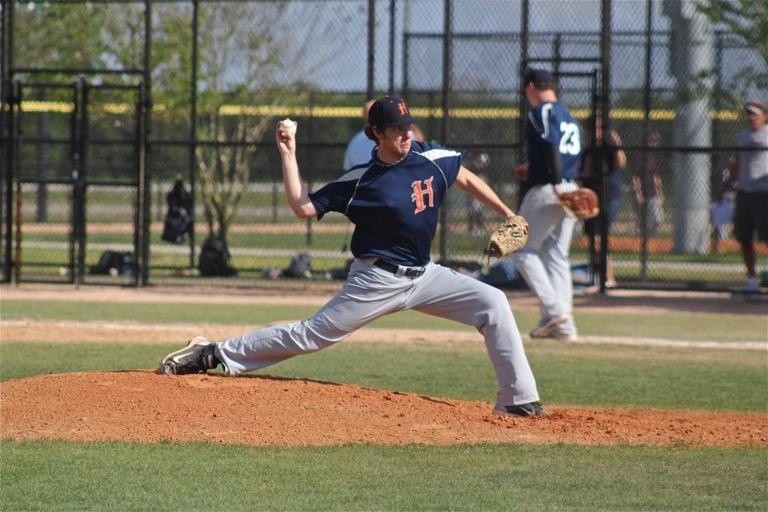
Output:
484;216;528;257
560;189;600;217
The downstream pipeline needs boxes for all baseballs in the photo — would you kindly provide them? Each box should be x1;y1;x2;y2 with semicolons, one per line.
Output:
278;119;297;135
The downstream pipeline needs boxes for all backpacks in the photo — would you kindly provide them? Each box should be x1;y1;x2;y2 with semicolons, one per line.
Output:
261;253;313;279
199;233;237;278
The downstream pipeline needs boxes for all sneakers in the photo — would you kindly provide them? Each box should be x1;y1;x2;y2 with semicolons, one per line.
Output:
530;315;575;339
162;336;217;375
492;402;543;416
729;277;767;295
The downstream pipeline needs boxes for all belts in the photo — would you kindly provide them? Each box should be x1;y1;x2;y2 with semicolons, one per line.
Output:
374;259;427;277
561;176;578;183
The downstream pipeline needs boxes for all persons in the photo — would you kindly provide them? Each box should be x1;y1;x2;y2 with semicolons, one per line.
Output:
510;70;586;338
465;132;489;237
457;257;597;287
160;97;543;417
199;221;238;276
709;155;736;241
631;129;665;235
578;113;626;296
344;100;425;172
727;98;767;293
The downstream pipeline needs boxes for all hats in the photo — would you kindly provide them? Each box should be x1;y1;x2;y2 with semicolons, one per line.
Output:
368;96;416;126
525;70;554;80
745;99;767;115
